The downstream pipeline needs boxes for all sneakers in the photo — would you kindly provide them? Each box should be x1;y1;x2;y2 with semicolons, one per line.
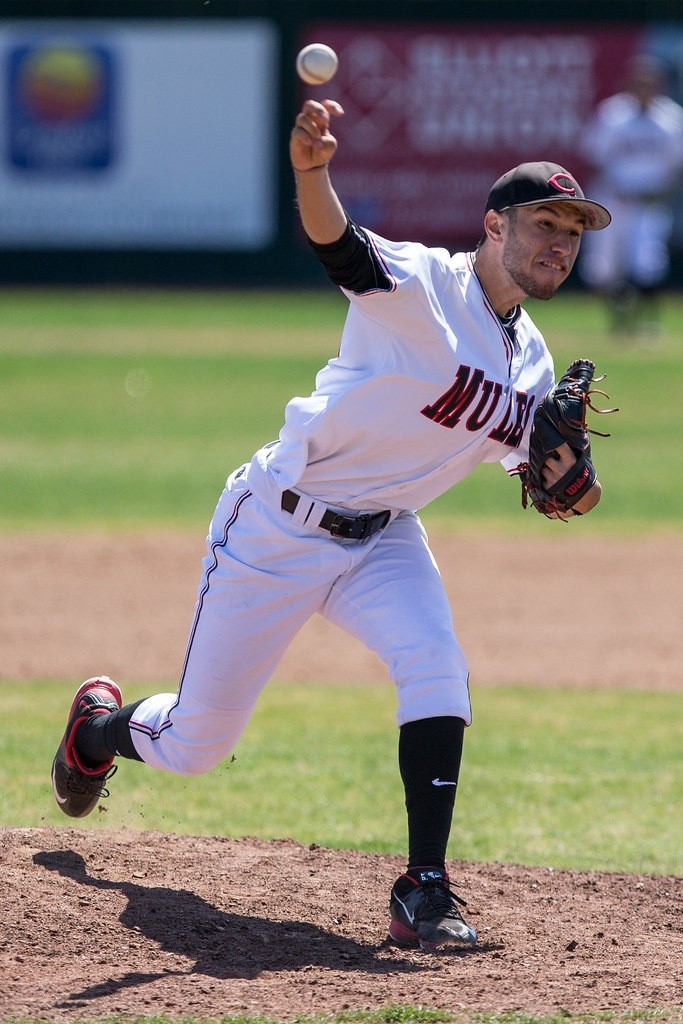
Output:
388;866;478;951
50;676;124;819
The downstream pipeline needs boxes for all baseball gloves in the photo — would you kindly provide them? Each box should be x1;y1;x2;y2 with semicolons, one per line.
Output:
507;356;621;523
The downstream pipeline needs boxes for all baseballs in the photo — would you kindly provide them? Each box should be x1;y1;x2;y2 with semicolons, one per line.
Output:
294;42;340;88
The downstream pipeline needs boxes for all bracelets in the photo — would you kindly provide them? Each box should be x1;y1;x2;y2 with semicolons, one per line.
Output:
293;164;330;172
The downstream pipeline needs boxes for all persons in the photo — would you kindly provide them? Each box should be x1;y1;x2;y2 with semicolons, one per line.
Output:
579;53;683;312
50;99;611;959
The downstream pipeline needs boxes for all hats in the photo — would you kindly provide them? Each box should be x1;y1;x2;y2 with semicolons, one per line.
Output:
485;161;612;231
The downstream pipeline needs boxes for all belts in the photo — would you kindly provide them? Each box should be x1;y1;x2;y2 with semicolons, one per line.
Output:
280;488;391;540
